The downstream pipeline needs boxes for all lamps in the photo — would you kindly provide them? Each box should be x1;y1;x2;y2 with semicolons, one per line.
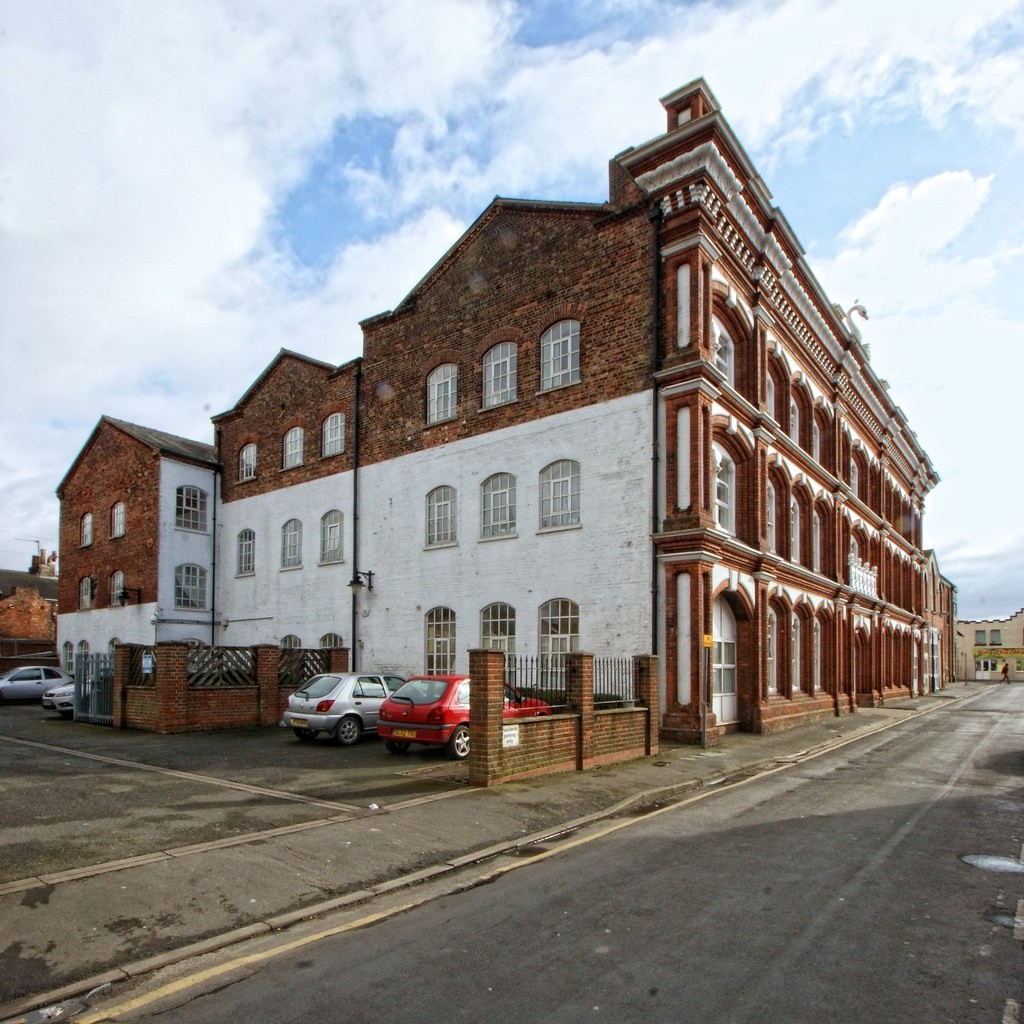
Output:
347;568;374;595
117;585;142;607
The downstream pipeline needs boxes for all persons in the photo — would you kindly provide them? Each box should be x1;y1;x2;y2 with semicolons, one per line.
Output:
998;663;1012;684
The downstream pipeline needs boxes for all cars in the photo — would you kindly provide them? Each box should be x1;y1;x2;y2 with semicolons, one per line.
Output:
41;666;114;718
278;670;439;748
0;665;76;701
374;674;554;760
